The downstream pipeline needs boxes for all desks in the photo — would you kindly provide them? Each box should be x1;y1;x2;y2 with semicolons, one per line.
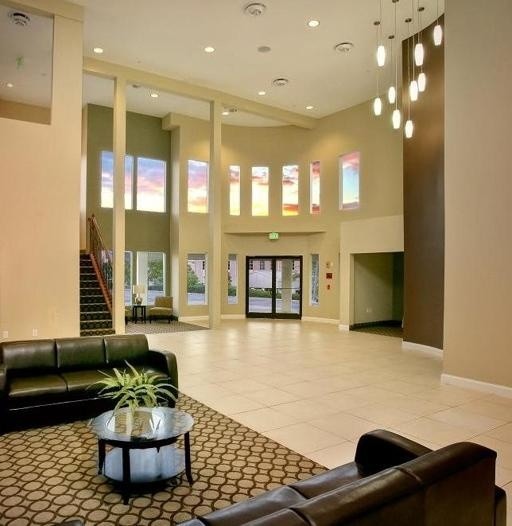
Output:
133;306;147;323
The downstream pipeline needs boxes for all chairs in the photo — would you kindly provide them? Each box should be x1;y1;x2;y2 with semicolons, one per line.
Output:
149;296;174;325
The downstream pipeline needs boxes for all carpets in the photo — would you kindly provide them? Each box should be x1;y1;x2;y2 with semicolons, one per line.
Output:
125;319;213;336
0;397;330;525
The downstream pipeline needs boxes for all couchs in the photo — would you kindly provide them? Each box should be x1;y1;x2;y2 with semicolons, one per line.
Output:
0;332;182;433
177;425;508;526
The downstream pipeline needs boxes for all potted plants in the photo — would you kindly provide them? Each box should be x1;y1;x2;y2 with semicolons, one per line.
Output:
83;360;179;434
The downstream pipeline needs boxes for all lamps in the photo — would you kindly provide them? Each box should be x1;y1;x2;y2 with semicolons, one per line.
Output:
132;285;145;304
372;0;444;142
